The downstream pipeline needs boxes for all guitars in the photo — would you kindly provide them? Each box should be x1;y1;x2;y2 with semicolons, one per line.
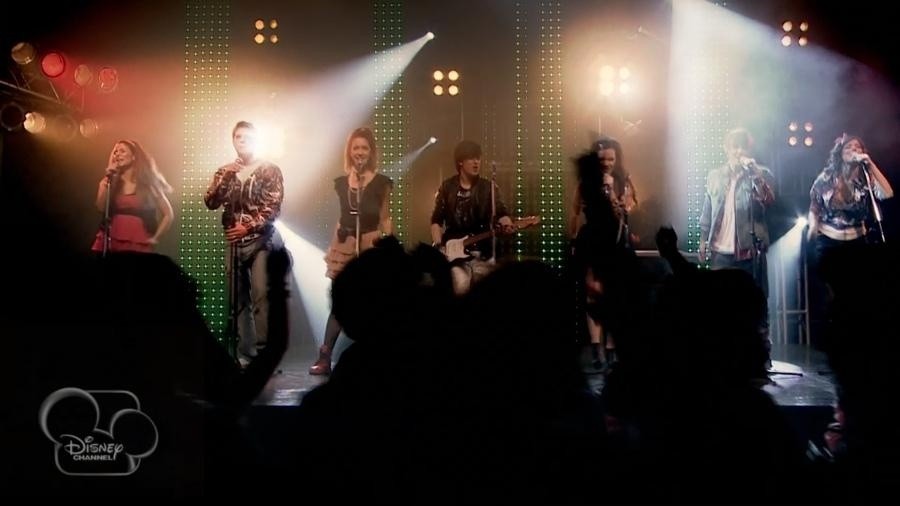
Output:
437;215;539;265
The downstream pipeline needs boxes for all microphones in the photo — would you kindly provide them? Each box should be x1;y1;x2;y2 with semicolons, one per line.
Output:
490;161;497;181
740;155;755;170
106;162;119;177
849;151;870;166
355;156;364;171
226;157;243;181
603;172;611;196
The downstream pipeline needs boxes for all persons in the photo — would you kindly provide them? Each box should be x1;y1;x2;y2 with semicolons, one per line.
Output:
806;134;895;274
566;137;641;369
203;121;284;378
304;127;395;376
88;139;175;255
695;129;779;368
430;139;510;268
2;235;899;506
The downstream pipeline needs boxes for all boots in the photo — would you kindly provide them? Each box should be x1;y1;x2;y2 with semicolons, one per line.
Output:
309;345;333;374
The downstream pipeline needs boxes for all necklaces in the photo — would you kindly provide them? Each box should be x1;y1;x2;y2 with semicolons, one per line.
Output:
347;173;364;211
